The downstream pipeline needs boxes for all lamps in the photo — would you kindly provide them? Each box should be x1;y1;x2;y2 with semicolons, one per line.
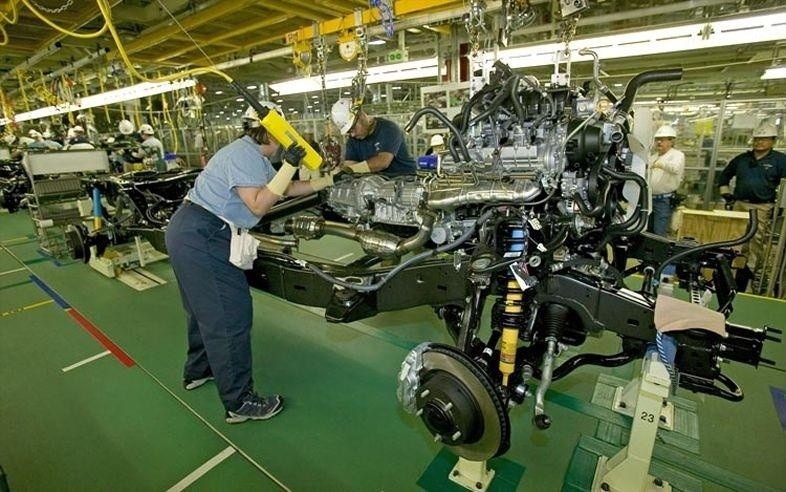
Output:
2;76;199;126
268;5;786;98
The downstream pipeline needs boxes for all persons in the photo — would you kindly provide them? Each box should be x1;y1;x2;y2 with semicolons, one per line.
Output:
6;114;165;162
165;102;331;424
333;98;444;179
647;126;684;237
716;123;784;292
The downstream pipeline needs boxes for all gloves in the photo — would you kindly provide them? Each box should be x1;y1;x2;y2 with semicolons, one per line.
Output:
333;171;347;182
284;141;307;168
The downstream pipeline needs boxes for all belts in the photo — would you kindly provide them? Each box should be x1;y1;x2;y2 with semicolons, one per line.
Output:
651;194;675;200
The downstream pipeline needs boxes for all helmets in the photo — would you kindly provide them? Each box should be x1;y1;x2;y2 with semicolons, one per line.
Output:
5;112;88;145
118;120;133;135
137;124;154;134
330;98;359;135
753;123;777;139
241;102;286;123
654;126;678;138
429;134;445;147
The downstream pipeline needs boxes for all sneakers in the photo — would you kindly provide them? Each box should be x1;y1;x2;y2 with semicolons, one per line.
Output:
185;368;214;390
225;395;285;423
